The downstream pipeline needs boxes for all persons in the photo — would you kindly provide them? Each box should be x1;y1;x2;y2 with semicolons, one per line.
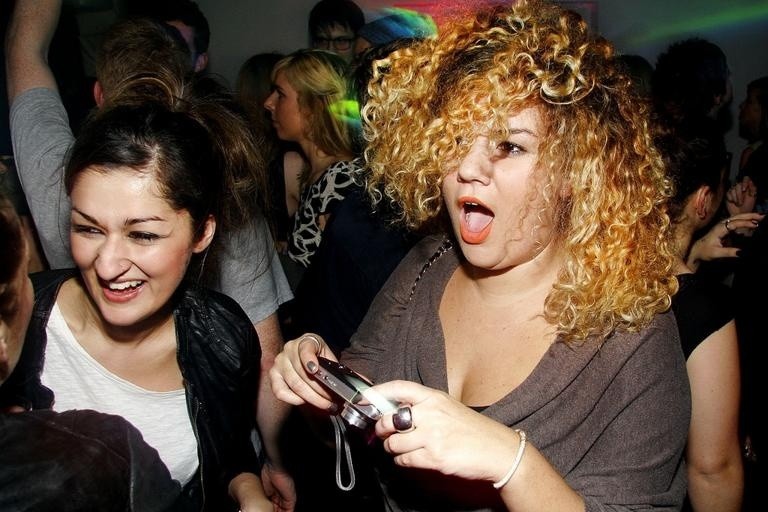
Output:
1;1;298;512
613;40;768;512
268;1;740;512
239;3;441;511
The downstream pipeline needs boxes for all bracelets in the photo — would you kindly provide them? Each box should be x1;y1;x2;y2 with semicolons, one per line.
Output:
493;429;525;490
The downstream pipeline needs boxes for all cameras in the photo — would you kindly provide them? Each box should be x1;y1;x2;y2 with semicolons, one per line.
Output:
313;356;400;430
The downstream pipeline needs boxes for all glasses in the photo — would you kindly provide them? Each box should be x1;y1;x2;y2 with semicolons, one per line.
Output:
313;34;356;53
